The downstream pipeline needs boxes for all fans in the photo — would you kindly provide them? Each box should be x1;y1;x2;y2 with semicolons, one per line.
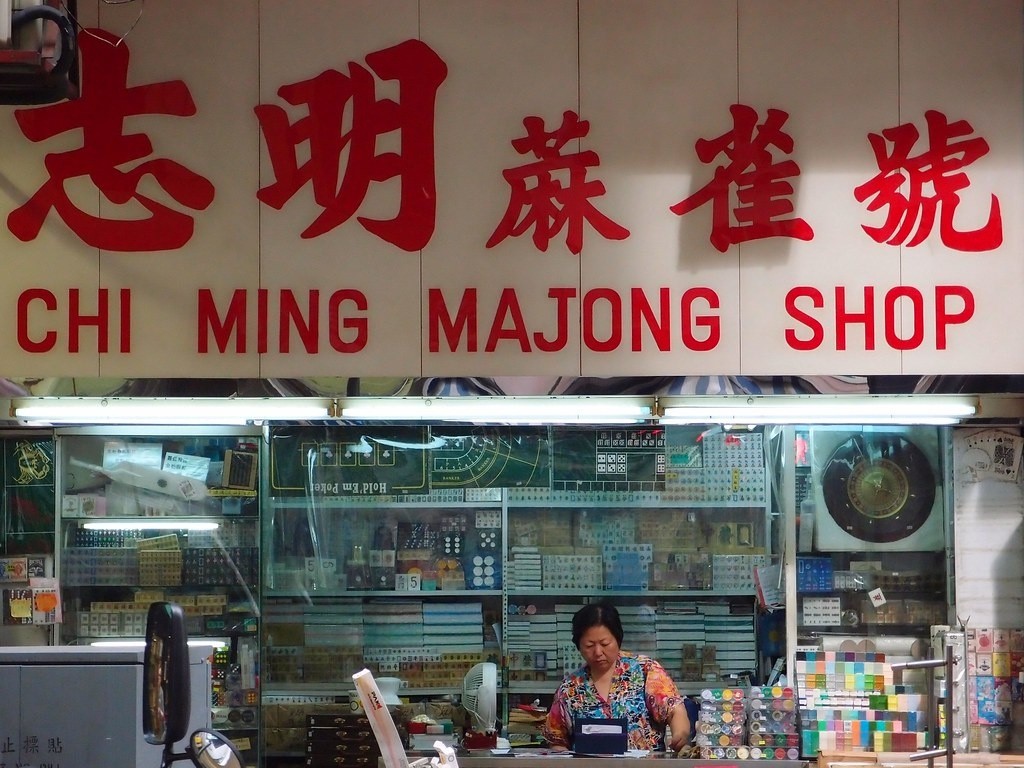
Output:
461;662;497;749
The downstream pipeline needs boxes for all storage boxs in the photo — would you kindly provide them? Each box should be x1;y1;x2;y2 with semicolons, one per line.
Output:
409;734;458;751
711;521;754;548
261;703;451;748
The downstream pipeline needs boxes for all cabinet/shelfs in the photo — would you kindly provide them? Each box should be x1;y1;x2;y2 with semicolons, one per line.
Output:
258;421;958;768
0;646;215;768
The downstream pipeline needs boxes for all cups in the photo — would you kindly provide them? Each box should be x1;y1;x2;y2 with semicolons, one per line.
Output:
348;690;364;714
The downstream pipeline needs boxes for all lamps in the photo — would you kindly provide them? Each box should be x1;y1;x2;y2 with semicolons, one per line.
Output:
6;394;983;425
77;519;224;530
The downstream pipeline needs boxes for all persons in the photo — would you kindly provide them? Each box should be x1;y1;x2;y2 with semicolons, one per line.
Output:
541;601;691;752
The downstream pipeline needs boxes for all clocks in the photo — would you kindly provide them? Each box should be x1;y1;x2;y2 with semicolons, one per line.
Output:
821;432;934;543
